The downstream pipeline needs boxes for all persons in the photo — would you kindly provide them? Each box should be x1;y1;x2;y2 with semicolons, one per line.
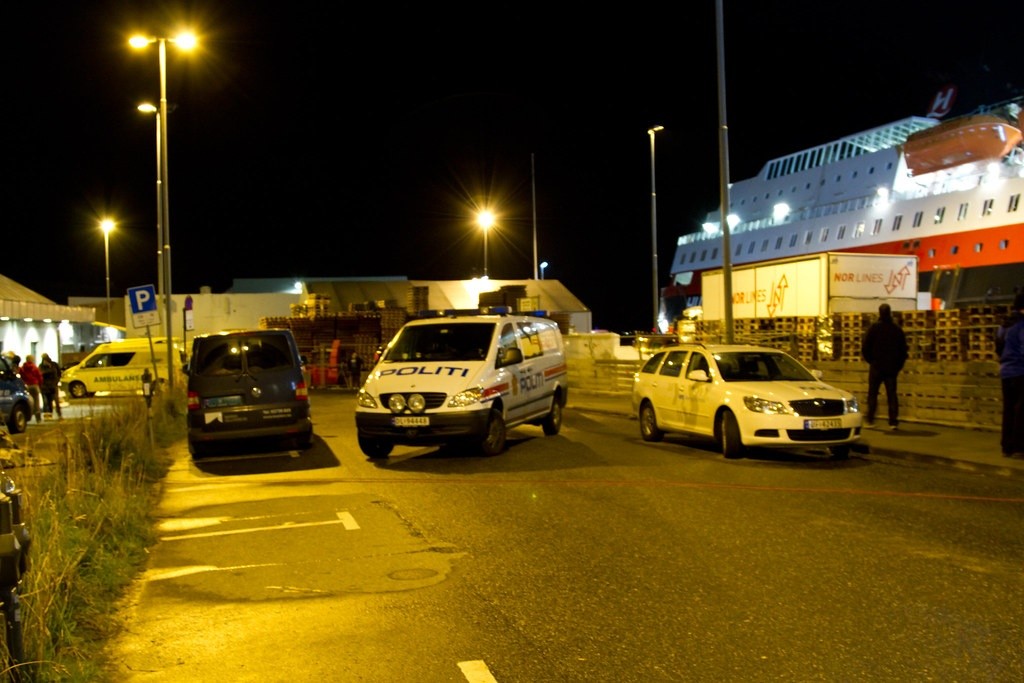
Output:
37;353;64;421
0;351;15;371
9;355;32;386
40;360;59;422
373;345;382;366
347;351;364;389
860;304;909;430
994;292;1024;459
20;353;45;424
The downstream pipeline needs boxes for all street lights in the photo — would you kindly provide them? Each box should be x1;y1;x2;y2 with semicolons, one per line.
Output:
646;124;663;333
125;26;201;405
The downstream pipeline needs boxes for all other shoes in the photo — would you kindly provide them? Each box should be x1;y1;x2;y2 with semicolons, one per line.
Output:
862;421;878;429
889;425;900;431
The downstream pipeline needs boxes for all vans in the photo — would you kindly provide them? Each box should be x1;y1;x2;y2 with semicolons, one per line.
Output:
354;308;572;460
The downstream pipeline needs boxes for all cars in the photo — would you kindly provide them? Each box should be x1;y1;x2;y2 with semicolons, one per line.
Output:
630;338;866;464
182;324;313;467
59;337;183;397
